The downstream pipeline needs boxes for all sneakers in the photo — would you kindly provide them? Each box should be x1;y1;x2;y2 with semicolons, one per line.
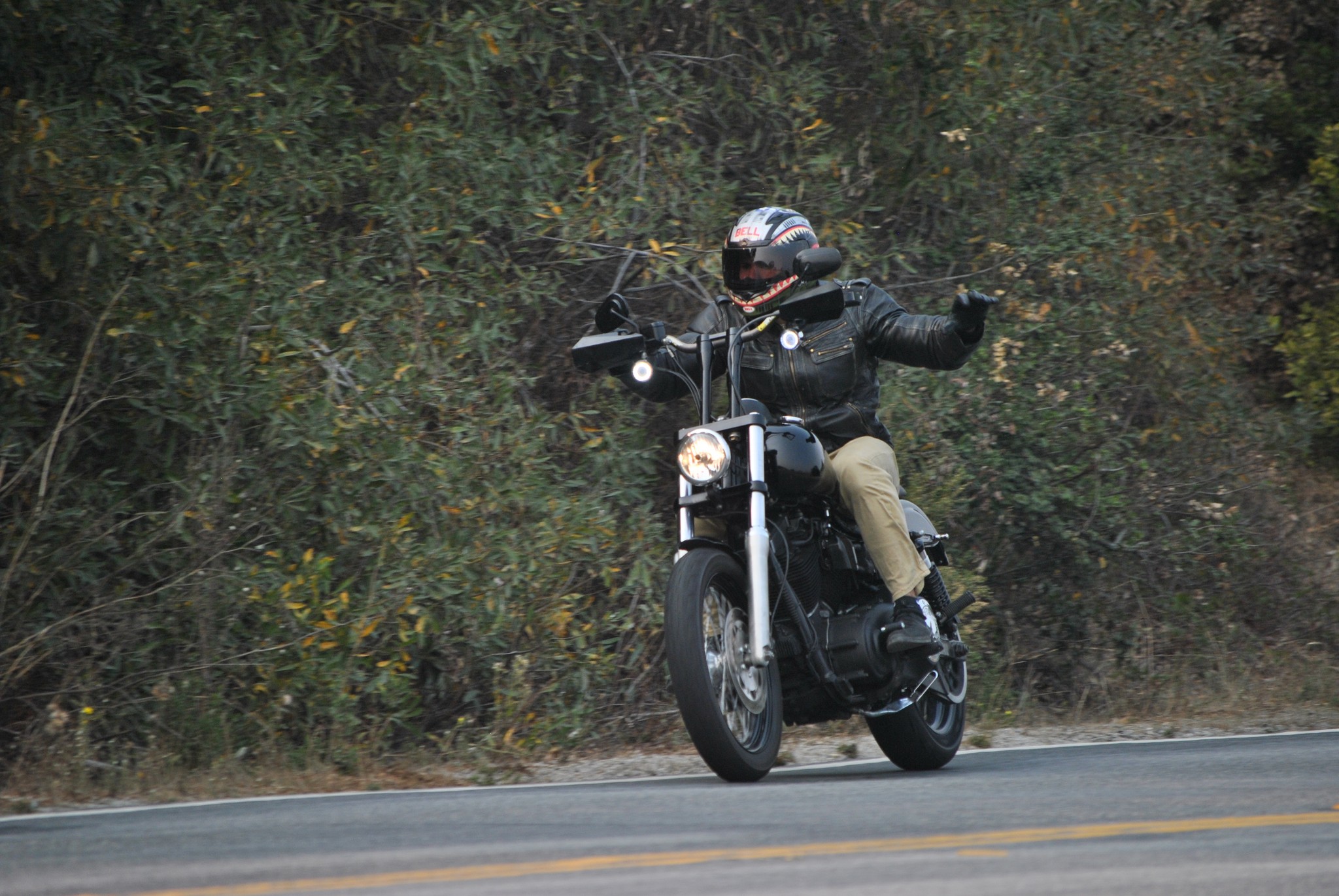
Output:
887;598;941;653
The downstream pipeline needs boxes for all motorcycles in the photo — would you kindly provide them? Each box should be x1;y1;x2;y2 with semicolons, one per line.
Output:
570;246;980;786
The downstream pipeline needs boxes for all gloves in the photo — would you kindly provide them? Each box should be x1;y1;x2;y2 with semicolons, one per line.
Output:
952;289;999;335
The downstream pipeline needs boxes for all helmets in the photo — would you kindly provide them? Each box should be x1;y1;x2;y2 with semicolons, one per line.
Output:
721;206;818;316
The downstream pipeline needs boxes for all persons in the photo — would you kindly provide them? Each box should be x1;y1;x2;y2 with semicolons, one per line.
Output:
608;204;999;653
